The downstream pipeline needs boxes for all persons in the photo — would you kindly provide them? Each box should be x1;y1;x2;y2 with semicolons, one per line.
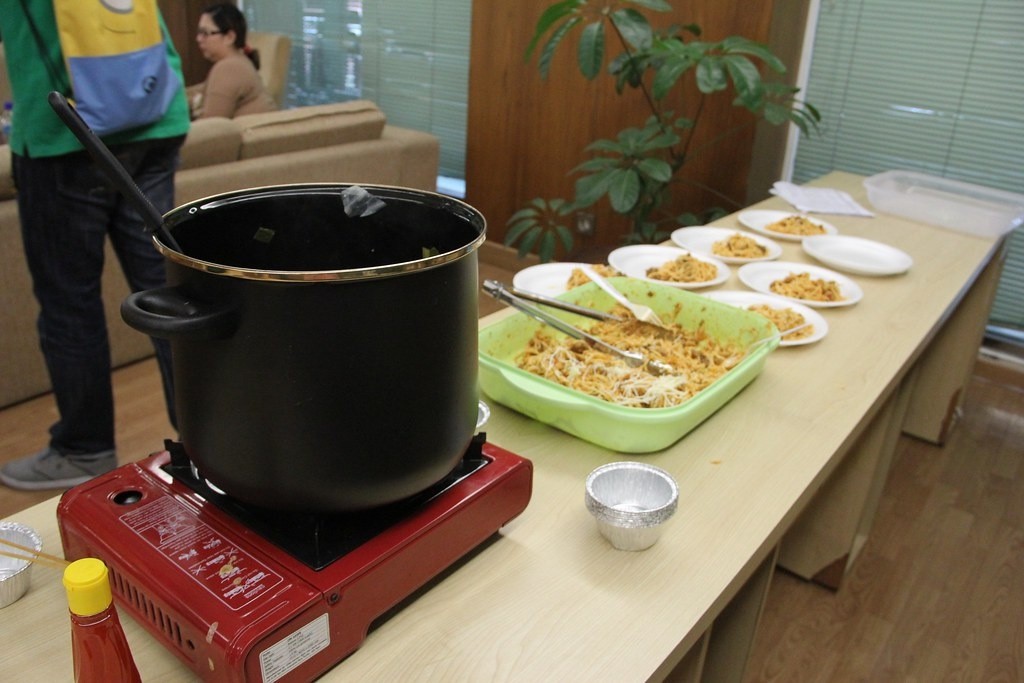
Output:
0;2;191;488
195;3;276;119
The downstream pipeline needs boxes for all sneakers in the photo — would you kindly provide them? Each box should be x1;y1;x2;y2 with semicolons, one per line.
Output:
1;444;117;489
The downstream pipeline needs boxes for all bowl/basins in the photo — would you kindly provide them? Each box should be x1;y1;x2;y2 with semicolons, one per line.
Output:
0;522;42;609
477;400;489;428
584;460;679;552
477;275;781;453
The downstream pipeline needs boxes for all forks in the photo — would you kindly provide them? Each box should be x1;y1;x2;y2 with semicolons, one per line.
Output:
580;261;666;327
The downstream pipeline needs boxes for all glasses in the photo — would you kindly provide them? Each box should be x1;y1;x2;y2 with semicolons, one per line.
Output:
196;28;225;37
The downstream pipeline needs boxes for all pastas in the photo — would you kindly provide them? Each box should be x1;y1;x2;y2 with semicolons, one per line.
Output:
508;305;745;409
569;215;844;345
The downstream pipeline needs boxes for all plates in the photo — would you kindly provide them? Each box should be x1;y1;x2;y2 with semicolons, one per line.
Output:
738;261;863;309
608;244;731;291
671;226;782;264
699;291;828;347
803;235;913;276
512;262;593;297
738;210;838;242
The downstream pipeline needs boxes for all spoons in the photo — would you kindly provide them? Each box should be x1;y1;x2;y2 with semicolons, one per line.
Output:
723;321;813;369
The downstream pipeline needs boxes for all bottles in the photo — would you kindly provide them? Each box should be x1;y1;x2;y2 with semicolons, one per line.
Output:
62;558;141;683
0;103;13;144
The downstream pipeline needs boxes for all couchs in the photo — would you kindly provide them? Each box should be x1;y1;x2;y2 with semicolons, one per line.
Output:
183;30;290;119
0;100;439;408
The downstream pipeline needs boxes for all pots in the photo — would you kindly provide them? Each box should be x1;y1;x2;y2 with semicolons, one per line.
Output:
120;182;489;513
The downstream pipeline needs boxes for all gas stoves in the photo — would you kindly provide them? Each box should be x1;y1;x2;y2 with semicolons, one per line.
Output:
57;430;533;683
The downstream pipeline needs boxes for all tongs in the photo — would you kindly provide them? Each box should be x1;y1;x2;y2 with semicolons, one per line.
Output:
482;277;675;379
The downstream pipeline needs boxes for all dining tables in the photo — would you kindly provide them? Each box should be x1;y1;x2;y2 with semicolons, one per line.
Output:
0;169;1018;683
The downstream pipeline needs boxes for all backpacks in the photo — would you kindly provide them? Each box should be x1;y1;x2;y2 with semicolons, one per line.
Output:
20;0;182;135
